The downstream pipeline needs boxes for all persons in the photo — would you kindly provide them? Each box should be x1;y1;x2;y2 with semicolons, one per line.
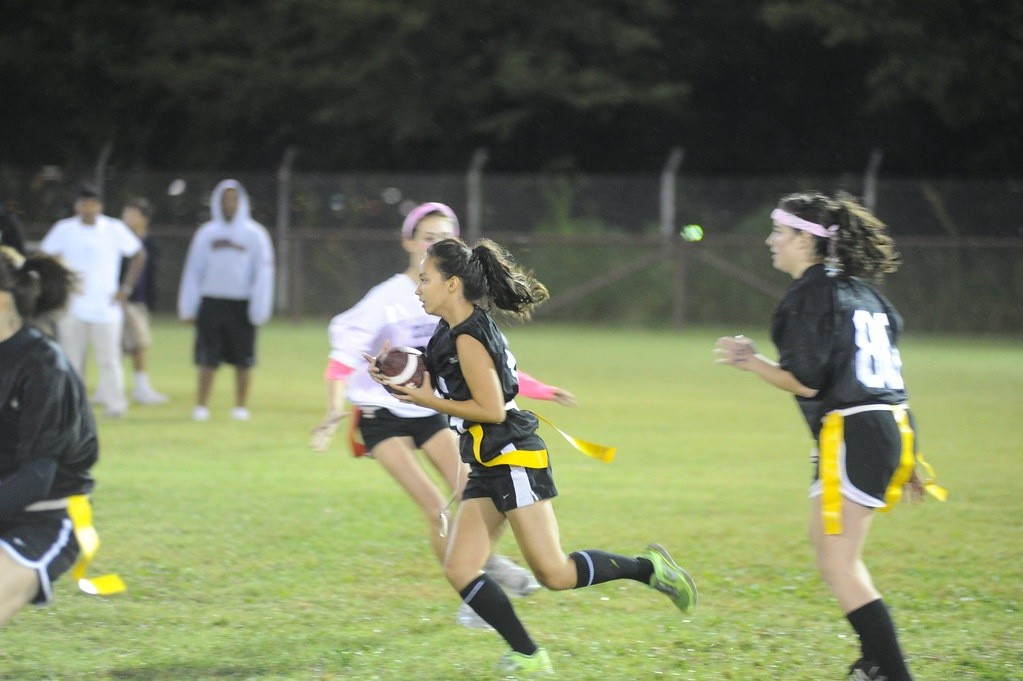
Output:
712;192;946;681
311;202;697;678
0;181;170;623
177;178;275;421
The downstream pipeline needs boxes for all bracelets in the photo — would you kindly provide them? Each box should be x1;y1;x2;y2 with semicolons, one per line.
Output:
121;284;134;297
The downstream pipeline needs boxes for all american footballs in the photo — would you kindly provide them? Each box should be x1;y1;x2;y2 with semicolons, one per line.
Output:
375;346;436;400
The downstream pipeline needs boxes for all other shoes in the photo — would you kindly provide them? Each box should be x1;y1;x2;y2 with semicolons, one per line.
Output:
235;407;246;419
134;387;164;405
194;405;211;419
848;658;888;681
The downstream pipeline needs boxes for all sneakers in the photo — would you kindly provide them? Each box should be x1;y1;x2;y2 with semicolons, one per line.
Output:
457;603;493;629
494;648;555;677
485;555;541;596
636;543;698;615
